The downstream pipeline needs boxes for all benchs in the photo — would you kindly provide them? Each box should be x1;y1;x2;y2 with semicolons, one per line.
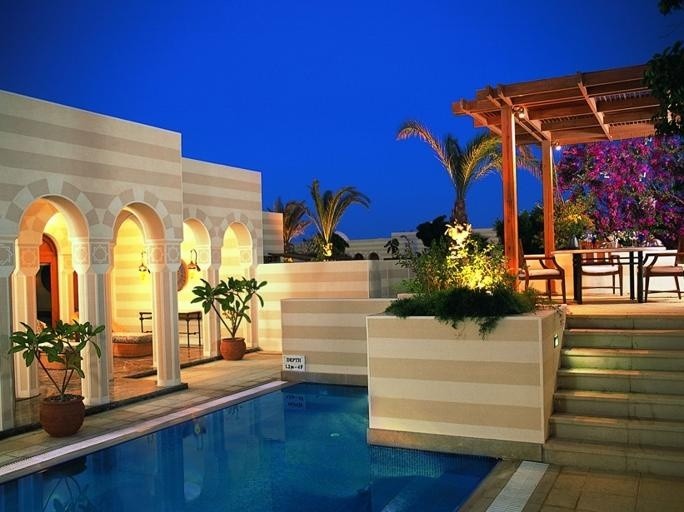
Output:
111;328;152;357
37;341;81;370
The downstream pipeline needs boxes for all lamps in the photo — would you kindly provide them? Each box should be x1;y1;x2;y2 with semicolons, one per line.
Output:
187;249;202;272
137;252;149;273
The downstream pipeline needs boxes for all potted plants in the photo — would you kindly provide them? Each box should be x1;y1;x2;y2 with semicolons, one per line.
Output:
6;316;107;440
188;275;266;363
40;454;95;511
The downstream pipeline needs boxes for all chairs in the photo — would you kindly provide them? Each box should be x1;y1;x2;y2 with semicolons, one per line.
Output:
518;237;567;304
639;226;684;302
577;238;624;297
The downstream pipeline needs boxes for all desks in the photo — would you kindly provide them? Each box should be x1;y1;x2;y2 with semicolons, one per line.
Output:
138;309;202;349
549;245;667;304
